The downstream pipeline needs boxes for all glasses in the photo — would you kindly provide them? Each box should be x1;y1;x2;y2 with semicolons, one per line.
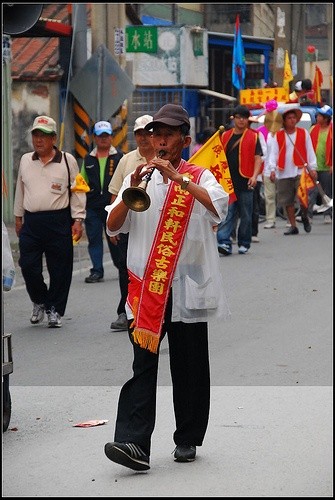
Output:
235;114;248;119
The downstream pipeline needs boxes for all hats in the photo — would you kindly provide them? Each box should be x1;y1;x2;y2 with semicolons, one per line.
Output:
144;103;190;132
31;115;57;136
133;115;153;132
93;121;111;136
275;106;303;124
316;104;332;116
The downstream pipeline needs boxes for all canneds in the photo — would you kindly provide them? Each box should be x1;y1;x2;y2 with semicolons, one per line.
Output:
3;269;15;291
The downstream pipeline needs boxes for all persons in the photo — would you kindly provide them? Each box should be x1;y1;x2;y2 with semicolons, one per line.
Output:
107;115;156;329
80;121;126;283
13;116;87;328
104;105;230;471
216;105;333;255
289;79;315;104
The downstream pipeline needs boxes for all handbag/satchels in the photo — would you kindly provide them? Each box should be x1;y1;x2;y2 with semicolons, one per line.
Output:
2;222;16;292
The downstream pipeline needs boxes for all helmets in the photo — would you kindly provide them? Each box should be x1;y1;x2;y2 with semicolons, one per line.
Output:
294;81;302;92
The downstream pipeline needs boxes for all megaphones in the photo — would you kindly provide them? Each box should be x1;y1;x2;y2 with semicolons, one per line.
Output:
3;3;43;34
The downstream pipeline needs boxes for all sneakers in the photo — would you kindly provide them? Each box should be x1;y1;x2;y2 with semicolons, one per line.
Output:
85;272;104;283
30;302;45;324
105;443;150;471
111;313;129;330
47;306;62;328
174;441;197;462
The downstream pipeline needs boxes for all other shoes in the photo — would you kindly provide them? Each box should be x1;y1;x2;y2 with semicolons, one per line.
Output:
252;236;260;243
302;215;311;232
238;246;248;254
323;215;331;224
264;224;275;229
217;244;232;255
284;227;299;236
296;215;312;224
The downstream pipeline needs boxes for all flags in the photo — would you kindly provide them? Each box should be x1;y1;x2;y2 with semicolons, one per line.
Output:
187;130;237;204
312;65;323;104
283;50;293;102
232;14;247;91
264;50;270;83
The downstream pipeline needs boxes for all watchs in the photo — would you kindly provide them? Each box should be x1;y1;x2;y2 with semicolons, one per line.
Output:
181;177;189;190
